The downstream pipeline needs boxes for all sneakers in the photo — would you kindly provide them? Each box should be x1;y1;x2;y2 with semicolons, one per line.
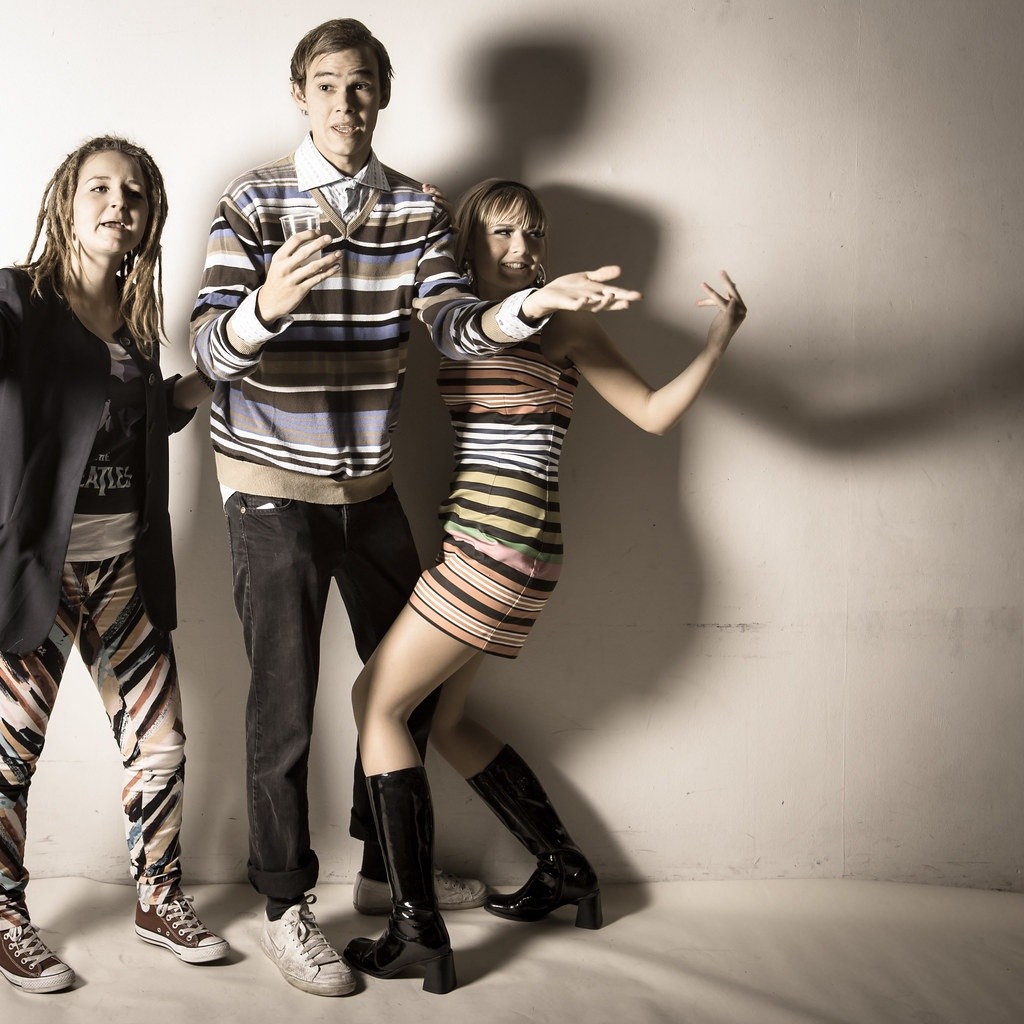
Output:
353;869;489;915
260;894;357;996
0;922;76;994
134;894;230;963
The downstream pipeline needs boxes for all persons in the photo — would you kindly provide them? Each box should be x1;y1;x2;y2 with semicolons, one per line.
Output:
343;173;747;995
180;15;642;999
0;132;231;999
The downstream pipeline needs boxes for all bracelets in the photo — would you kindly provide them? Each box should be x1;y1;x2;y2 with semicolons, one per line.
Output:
195;366;217;394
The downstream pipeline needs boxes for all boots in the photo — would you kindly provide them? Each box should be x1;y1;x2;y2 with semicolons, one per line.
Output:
465;744;598;929
344;766;456;995
